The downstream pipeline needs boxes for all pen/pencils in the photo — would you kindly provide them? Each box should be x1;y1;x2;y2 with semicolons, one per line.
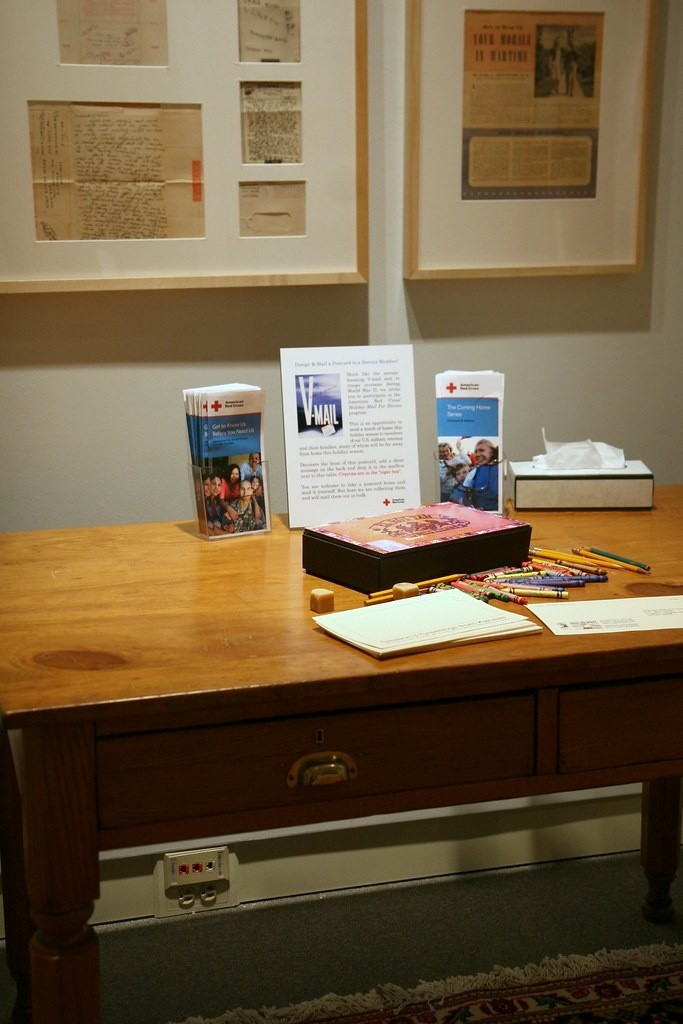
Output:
363;545;653;607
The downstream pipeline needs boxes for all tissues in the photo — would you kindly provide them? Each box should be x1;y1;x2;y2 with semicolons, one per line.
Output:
505;425;655;515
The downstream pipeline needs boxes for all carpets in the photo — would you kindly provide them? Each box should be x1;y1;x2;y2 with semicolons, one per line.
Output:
188;944;683;1024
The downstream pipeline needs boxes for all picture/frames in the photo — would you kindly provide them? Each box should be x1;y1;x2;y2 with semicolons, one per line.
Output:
400;0;662;281
0;1;374;297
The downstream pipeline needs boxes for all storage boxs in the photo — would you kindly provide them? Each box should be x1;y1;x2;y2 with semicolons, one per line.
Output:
300;498;535;597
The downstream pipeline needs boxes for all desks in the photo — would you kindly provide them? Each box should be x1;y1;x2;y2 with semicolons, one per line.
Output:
0;482;682;1024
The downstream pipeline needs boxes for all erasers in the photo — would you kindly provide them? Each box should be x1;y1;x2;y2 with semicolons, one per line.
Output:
392;582;419;602
308;587;336;613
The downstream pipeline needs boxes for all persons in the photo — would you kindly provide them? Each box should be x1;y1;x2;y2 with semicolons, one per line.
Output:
240;453;262;480
463;438;498;510
451;463;471;505
202;473;232;535
218;464;242;520
438;442;473;503
249;475;265;515
220;480;266;533
